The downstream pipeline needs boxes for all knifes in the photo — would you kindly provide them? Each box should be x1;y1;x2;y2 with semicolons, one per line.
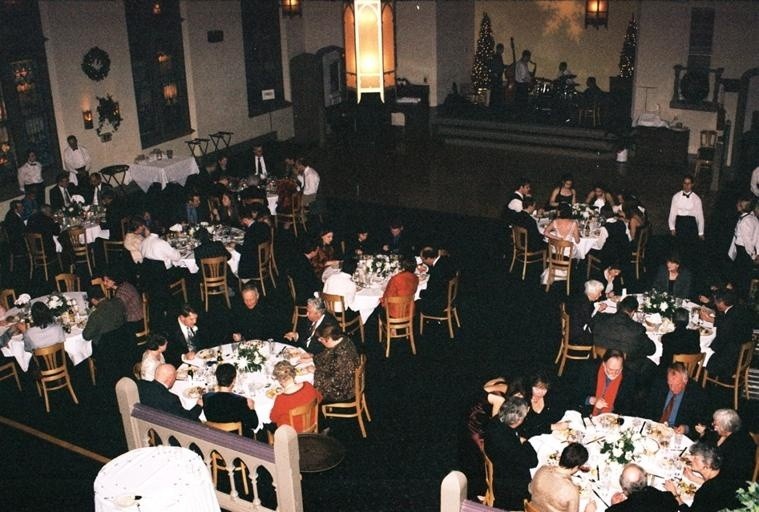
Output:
581;415;586;429
596;464;600;480
639;420;647;434
592;489;610;508
584;435;605;445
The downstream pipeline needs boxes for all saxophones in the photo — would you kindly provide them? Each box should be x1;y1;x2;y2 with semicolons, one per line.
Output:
526;59;537;92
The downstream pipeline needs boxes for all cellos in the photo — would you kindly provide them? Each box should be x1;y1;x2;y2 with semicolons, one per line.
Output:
504;37;521;109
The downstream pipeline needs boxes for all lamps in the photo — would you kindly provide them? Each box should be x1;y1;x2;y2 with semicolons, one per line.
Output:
583;0;610;31
82;109;94;130
281;0;303;19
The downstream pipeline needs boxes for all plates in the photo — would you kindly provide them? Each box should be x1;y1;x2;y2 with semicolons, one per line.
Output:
174;348;306;401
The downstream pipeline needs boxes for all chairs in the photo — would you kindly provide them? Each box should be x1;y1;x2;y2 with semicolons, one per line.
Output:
577;100;603;127
380;296;416;356
419;278;460;338
205;422;249;496
1;357;22;390
268;399;320;449
322;291;365;342
35;343;80;413
523;500;540;512
701;342;754;410
323;356;371;437
483;452;494;508
1;129;327;354
502;190;652;288
554;303;593;376
749;433;759;482
674;354;706;382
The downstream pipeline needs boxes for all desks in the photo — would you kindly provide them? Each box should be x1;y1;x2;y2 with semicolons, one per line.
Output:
174;341;315;432
532;416;707;512
325;252;430;324
596;293;717;364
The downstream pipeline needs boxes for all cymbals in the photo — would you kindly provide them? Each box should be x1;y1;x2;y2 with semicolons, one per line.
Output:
536;77;551;82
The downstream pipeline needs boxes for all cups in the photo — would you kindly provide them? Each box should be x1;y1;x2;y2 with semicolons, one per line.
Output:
647;473;655;486
617;414;625;426
674;433;683;449
659;435;671;449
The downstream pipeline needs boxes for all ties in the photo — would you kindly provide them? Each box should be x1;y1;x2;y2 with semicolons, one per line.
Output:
308;321;317;342
186;327;192;348
662;393;677;424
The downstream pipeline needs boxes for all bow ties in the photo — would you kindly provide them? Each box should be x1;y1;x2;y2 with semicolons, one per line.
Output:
683;193;691;198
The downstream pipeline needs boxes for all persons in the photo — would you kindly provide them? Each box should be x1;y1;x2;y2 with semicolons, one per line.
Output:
134;217;456;479
565;77;600;124
500;166;759;305
552;62;573;95
495;43;509;105
0;136;320;400
515;50;531;122
467;255;759;511
